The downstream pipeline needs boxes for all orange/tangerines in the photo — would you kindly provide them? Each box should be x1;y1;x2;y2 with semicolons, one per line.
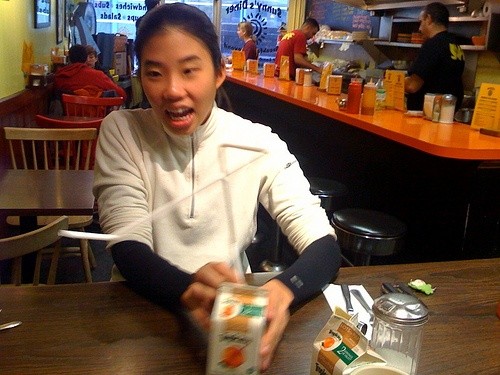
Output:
222;347;242;368
323;337;335;348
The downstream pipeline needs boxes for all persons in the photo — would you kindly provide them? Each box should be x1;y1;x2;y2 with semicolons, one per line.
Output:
404;2;465;115
274;17;321;81
92;3;342;370
227;22;259;64
135;0;160;35
54;44;127;99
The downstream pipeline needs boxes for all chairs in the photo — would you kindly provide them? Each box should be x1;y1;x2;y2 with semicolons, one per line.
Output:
0;93;123;285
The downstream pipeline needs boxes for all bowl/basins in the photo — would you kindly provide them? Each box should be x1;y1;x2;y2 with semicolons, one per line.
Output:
472;36;485;46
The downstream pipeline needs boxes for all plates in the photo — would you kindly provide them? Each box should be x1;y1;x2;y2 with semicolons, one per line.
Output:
352;31;368;40
397;32;425;44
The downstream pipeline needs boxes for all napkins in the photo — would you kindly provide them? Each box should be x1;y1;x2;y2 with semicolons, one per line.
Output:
322;283;374;319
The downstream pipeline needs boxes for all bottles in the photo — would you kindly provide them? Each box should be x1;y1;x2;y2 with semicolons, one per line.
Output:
370;293;431;375
438;94;456;123
346;75;363;114
432;96;441;122
361;78;376;116
295;67;313;87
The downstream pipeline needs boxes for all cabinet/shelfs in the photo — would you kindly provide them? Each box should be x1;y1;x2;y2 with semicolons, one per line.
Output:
373;0;492;51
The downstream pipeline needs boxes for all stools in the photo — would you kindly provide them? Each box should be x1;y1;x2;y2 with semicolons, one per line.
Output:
329;209;402;266
274;178;346;261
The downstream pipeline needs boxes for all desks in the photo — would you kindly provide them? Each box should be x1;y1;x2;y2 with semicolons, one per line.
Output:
0;168;94;283
0;257;500;375
